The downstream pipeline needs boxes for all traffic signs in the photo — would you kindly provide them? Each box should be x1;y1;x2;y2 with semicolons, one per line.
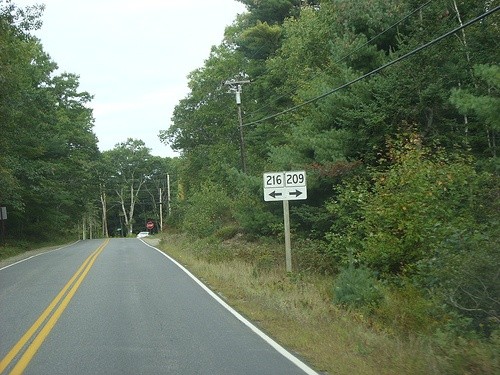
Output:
263;171;308;202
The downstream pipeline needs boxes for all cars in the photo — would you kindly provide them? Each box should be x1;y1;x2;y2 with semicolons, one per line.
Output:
137;232;150;238
